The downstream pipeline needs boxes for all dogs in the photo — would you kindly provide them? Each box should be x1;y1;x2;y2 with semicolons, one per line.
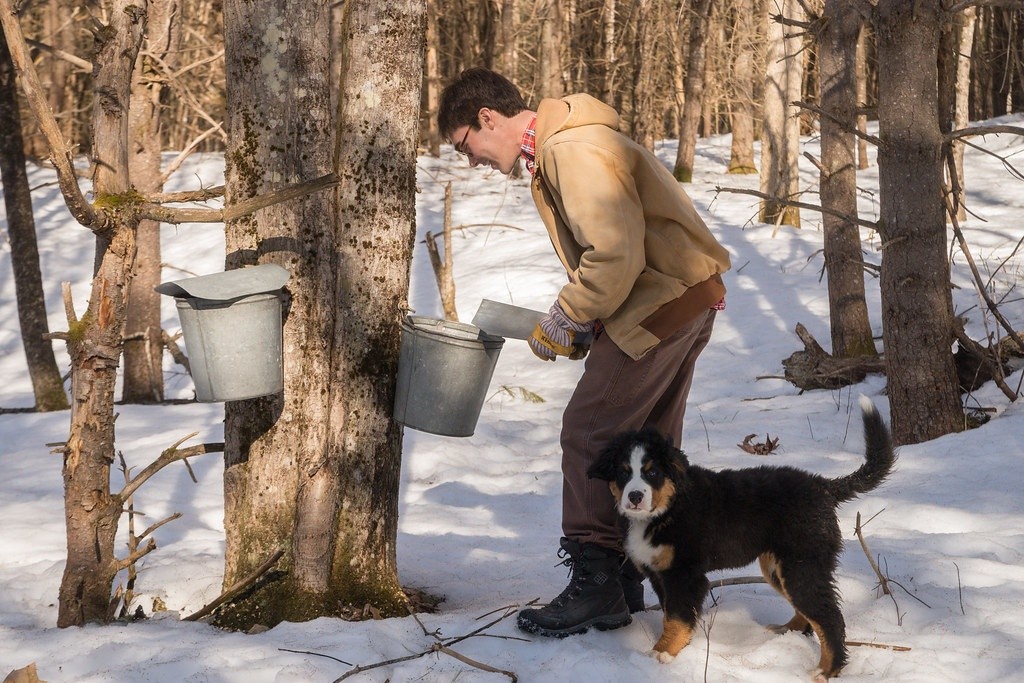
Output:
587;391;895;683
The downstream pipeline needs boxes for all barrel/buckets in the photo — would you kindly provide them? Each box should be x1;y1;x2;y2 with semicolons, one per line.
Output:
390;301;506;436
174;289;283;403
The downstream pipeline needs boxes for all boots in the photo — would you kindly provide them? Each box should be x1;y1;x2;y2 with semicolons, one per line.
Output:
516;536;633;639
617;549;646;612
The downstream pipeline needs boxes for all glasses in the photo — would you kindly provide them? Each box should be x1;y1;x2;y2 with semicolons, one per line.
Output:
457;124;471;156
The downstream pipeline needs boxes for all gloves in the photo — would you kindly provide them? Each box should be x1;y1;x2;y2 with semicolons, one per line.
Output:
527;298;595;361
569;319;604;360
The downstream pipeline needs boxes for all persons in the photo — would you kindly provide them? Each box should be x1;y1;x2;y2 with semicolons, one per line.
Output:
438;67;730;641
